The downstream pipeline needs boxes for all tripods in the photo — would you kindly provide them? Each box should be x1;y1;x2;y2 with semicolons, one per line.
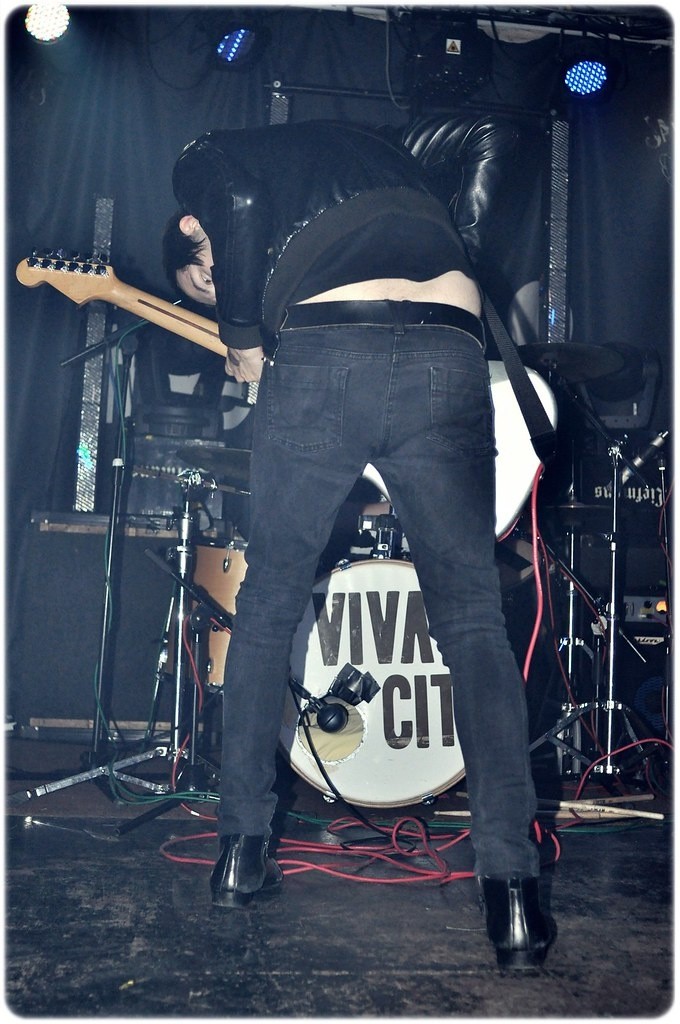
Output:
528;355;672;797
6;306;326;841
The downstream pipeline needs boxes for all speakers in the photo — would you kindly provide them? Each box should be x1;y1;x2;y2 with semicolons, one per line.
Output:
6;511;193;743
612;621;672;792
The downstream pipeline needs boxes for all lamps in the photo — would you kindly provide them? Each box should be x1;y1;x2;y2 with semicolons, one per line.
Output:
193;7;272;71
408;8;494;105
556;14;622;108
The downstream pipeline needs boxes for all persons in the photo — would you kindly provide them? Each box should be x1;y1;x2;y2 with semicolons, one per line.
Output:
162;110;559;973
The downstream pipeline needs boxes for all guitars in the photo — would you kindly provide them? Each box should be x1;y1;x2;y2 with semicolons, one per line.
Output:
14;247;559;546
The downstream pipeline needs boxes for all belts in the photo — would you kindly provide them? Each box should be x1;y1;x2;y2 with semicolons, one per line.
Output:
279;299;485;355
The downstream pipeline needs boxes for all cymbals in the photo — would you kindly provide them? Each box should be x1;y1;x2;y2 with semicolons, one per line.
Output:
176;445;252;492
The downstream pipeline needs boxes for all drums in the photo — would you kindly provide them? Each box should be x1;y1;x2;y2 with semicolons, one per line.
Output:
274;559;468;810
161;533;248;697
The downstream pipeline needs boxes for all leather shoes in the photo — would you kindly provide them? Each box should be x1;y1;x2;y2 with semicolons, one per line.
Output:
482;878;558;975
208;834;284;909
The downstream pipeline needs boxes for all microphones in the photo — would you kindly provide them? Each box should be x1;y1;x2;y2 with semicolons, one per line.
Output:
316;705;349;734
609;429;669;494
